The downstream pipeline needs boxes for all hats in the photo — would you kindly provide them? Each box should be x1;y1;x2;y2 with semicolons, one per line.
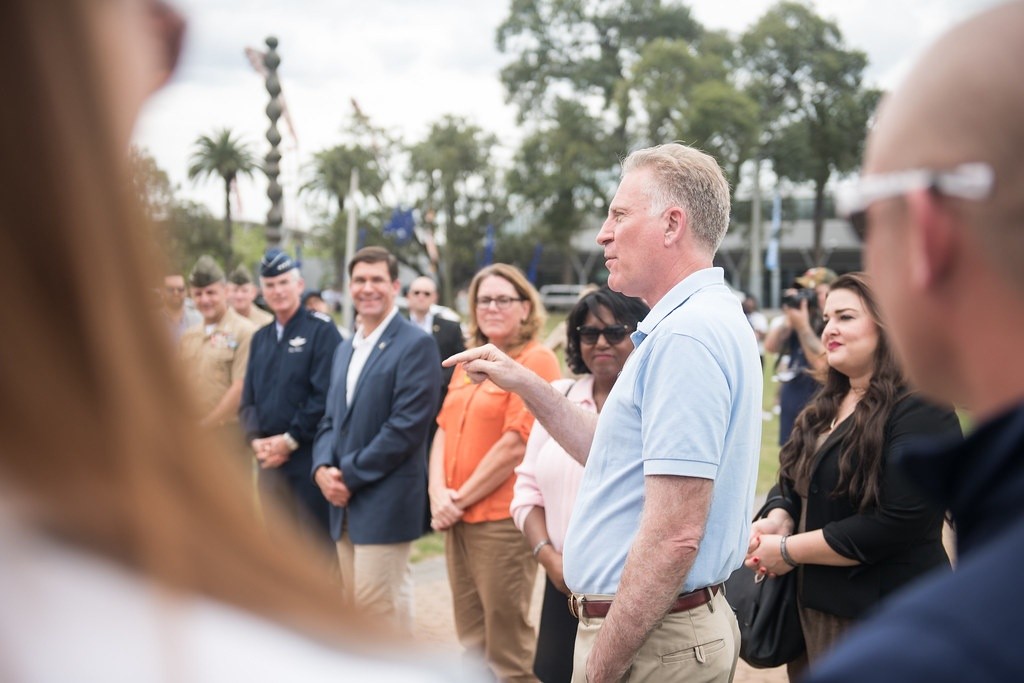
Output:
258;248;300;278
793;267;838;289
228;262;255;285
187;253;223;286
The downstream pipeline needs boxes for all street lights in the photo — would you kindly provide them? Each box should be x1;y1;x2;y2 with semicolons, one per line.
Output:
731;155;778;308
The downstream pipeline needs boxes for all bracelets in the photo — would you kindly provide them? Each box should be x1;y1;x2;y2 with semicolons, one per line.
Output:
532;541;550;557
283;432;298;450
782;536;801;567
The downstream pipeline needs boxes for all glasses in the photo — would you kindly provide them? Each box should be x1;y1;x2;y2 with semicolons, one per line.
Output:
166;285;185;294
575;325;635;345
476;296;526;312
825;158;999;241
411;289;436;298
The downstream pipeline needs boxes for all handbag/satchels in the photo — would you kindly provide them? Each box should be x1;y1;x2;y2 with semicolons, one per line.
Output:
722;495;797;670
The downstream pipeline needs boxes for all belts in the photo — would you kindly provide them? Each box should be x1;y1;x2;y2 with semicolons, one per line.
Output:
567;584;721;618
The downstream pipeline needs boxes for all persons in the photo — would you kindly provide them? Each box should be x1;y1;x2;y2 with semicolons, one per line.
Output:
430;262;560;683
745;272;964;675
313;246;442;619
800;0;1024;683
404;275;465;393
301;290;328;313
744;266;837;446
511;288;650;683
441;142;763;682
157;275;201;341
226;264;274;328
181;254;254;504
239;248;340;549
0;1;480;683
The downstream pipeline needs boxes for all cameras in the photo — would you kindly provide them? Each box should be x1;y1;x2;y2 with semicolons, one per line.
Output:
784;287;818;310
